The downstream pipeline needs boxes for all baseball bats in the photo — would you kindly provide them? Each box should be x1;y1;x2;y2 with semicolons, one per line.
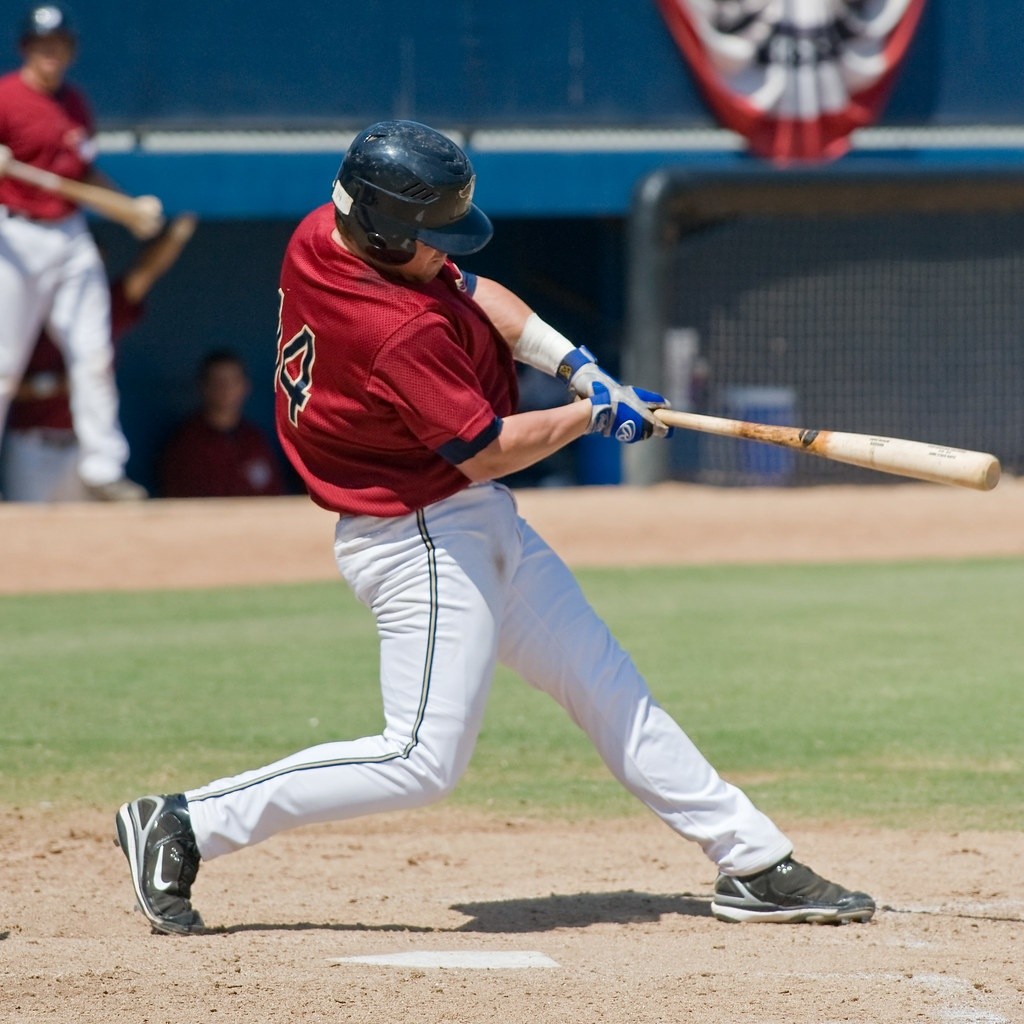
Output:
573;392;1004;492
6;159;147;223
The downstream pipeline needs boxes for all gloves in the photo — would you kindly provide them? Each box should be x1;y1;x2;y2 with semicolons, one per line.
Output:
588;385;676;444
555;344;619;399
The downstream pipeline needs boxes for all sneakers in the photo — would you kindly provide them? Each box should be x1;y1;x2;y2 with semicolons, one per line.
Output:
712;857;879;925
117;790;206;939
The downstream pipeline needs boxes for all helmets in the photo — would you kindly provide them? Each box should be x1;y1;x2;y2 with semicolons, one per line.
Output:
21;7;83;47
330;119;493;264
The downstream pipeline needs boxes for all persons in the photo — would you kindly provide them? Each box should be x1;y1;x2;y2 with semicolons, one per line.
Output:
160;346;283;498
0;6;197;499
117;120;875;937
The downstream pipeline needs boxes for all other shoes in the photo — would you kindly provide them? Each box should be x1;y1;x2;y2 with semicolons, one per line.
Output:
89;478;142;505
